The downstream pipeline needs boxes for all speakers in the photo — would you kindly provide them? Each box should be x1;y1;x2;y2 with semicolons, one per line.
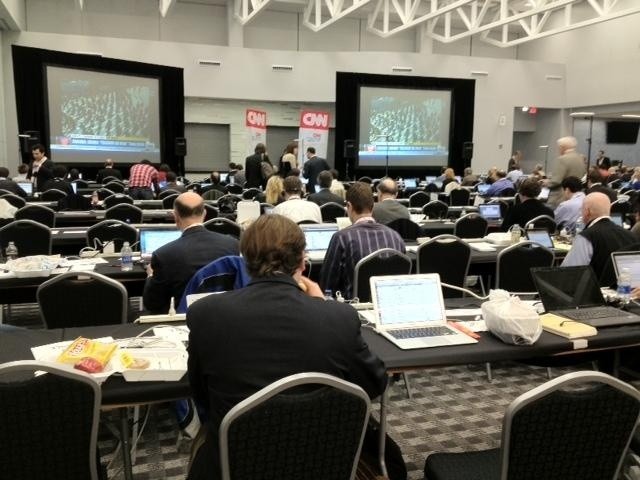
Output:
23;130;42;154
343;139;357;160
462;141;474;160
174;137;188;156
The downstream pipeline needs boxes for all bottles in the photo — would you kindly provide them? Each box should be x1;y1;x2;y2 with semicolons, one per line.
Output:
5;242;17;261
121;242;133;273
575;216;585;237
617;268;632;306
459;209;467;219
510;223;521;248
324;289;335;302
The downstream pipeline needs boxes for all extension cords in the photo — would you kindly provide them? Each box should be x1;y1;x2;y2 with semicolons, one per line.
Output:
352;301;374;311
138;311;186;324
80;249;101;257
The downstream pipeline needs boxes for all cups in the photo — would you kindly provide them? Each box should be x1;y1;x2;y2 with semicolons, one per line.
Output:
152;324;176;340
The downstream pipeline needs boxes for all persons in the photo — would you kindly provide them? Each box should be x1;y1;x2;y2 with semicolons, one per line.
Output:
159;172;188;194
330;168;346;201
302;147;330;193
159;163;170;183
435;168;446;183
486;169;515;198
542;135;588;191
371;176;411;225
26;143;55;192
281;142;301;176
320;181;406;300
253;176;287;214
595;149;611;171
127;159;161;200
201;171;229;196
553;176;588;230
506;164;523;182
234;164;246;185
44;164;73;196
97;158;121;183
559;191;640;289
142;191;240;315
273;175;324;224
185;213;407;480
500;176;556;235
507;150;521;172
461;167;476;185
0;166;28;199
306;170;345;208
586;170;618;202
226;162;236;183
12;163;29;180
245;143;273;188
441;167;459;192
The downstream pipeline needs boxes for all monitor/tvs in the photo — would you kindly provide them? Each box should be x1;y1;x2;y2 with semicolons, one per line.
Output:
219;173;229;182
426;175;437;184
610;212;623;228
404;178;416;188
139;226;182;260
610;250;640;289
299;224;340;261
478;203;502;220
16;182;34;196
477;184;492;193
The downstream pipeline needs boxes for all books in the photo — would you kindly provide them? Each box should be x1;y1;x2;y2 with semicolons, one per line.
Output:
469;242;498;253
540;312;599;339
58;337;117;367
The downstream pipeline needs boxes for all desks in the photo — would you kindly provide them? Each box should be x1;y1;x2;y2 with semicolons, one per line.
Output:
405;203;478;217
89;184;106;188
398;230;585;291
1;249;180;301
134;199;164;209
1;279;639;479
51;225;94;249
415;219;505;235
49;225;98;244
77;187;102;193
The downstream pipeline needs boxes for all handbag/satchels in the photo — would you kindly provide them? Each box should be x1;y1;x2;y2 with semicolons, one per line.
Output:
260;153;273;180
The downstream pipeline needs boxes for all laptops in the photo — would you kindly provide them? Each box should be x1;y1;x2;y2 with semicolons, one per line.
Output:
529;264;640;328
368;272;479;351
525;227;560;251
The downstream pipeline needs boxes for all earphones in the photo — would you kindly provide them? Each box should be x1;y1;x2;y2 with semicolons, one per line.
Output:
303;257;312;278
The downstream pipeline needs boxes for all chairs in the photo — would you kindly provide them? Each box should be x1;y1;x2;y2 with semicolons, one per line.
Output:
0;188;17;199
220;371;390;480
201;189;222;198
0;219;52;256
607;178;622;188
159;189;180;199
597;240;640;300
417;234;471;297
38;188;66;201
495;240;554;295
71;179;88;187
103;193;133;211
94;188;114;202
421;200;449;219
425;371;640;479
85;219;139;251
104;182;124;193
387;218;424;242
524;215;556;234
35;271;128;328
408;190;430;208
260;201;274;214
14;205;55;227
444;181;459;197
353;248;412;304
610;199;635;214
450;187;469;206
453;211;488;238
314;201;347;222
101;176;122;183
1;194;25;207
202;217;240;237
104;203;142;224
163;194;179;209
205;204;218;221
485;199;508;217
0;360;102;480
184;258;257;310
